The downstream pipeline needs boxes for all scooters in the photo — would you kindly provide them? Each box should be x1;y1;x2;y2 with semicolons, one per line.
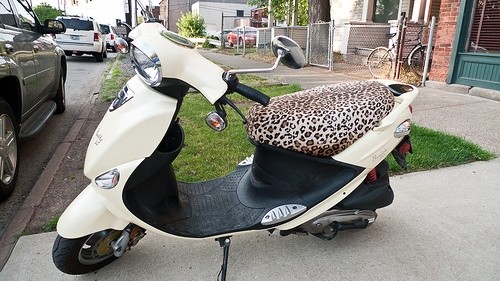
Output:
52;0;418;281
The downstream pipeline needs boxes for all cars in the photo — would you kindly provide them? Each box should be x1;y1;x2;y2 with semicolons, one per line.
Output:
214;29;232;42
227;26;256;47
100;24;118;52
51;16;107;62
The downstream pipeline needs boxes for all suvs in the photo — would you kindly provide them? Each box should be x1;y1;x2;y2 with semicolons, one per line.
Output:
0;0;67;202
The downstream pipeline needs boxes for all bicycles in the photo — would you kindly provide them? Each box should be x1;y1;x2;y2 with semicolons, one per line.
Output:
367;25;435;79
